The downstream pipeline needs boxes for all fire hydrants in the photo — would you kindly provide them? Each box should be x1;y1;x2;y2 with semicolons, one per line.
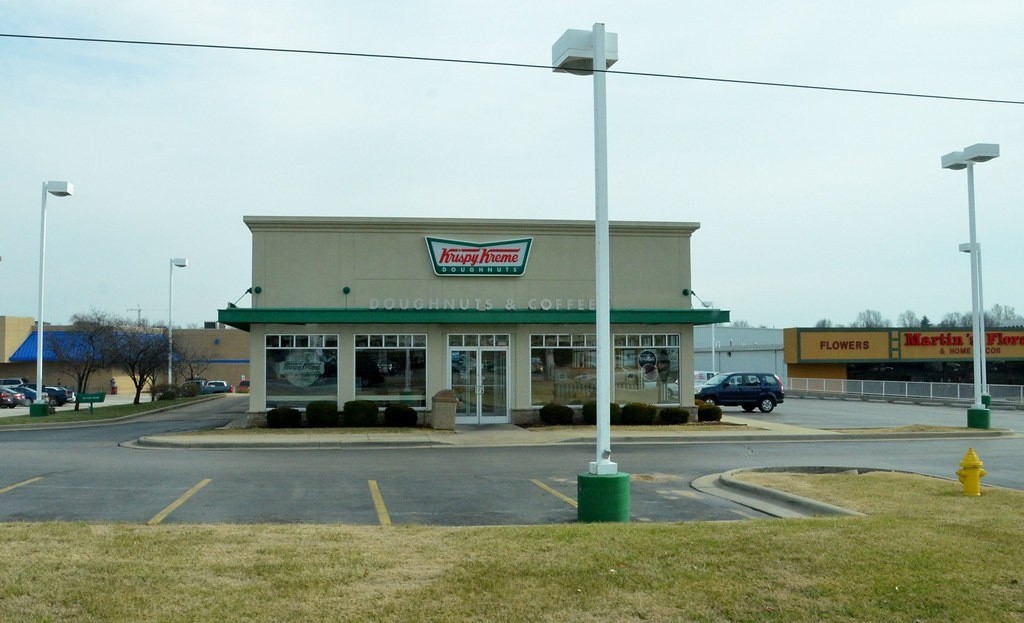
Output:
956;447;988;497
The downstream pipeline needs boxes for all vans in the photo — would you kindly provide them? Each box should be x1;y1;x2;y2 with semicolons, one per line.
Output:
0;378;25;390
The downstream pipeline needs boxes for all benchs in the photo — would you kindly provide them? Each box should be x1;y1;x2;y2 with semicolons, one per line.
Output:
753;382;761;385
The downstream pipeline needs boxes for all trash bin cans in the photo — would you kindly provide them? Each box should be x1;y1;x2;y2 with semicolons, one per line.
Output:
431;388;459;430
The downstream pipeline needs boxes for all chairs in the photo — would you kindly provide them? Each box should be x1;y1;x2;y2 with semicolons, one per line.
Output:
737;378;742;386
731;378;735;385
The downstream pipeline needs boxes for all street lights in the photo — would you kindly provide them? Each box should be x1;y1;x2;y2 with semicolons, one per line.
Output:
551;23;631;524
30;181;75;415
958;242;992;409
168;257;189;384
702;301;715;371
940;144;1000;431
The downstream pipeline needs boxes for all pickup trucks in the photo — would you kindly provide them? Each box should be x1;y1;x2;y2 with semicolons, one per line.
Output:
17;383;75;407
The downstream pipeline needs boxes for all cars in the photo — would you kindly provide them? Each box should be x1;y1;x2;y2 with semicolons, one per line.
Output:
237;379;250;394
693;372;785;414
451;356;491;372
62;387;76;403
575;365;641;390
316;354;385;389
373;357;400;375
0;388;26;409
206;381;227;386
666;379;706;398
8;387;50;407
531;358;545;374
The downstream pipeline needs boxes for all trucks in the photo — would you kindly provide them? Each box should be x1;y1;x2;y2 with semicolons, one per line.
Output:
179;380;235;395
